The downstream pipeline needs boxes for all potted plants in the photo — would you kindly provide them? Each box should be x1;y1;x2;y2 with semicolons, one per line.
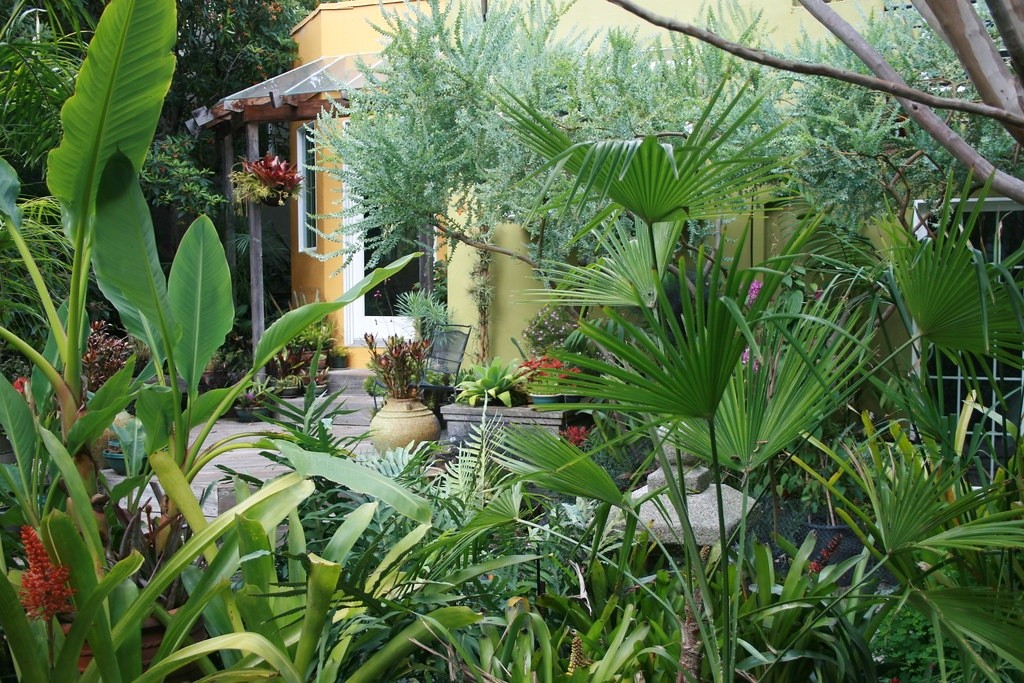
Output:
360;328;446;457
326;343;352;369
516;356;584;410
222;150;308;221
295;319;339;371
201;347;232;390
300;365;332;397
271;372;301;399
232;376;275;422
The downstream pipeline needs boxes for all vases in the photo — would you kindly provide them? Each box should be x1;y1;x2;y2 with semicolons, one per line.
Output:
102;439;154;475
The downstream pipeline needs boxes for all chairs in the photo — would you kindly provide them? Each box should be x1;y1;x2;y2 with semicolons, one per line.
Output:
362;322;473;412
